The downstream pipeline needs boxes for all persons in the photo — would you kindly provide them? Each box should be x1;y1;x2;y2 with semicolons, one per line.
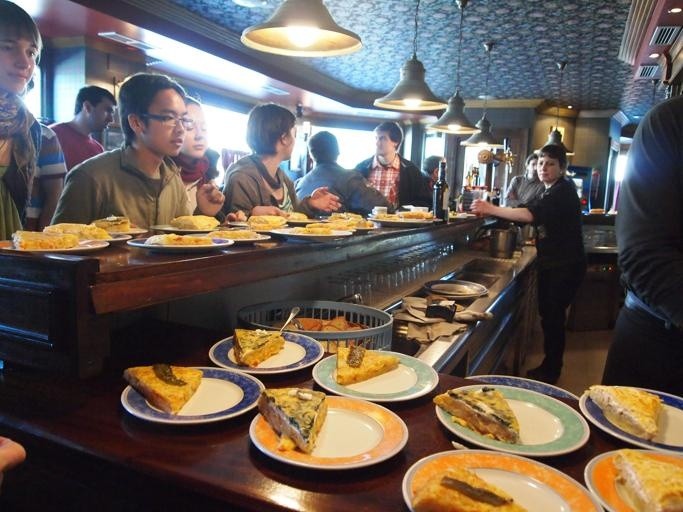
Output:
423;157;445;182
354;121;433;212
504;155;545;257
50;73;225;224
470;144;586;385
171;97;248;226
221;104;342;219
48;86;118;170
1;1;67;231
601;92;683;395
294;132;394;214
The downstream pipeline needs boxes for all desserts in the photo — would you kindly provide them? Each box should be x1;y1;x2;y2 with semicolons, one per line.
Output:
11;212;374;251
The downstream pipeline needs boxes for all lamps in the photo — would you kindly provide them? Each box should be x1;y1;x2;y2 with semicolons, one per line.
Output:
239;0;362;59
459;40;504;148
374;1;448;111
534;59;576;157
424;0;481;136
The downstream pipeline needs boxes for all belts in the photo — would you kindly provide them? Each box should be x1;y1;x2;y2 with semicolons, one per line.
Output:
622;290;674;330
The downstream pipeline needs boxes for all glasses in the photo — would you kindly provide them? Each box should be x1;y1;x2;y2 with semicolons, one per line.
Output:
137;111;192;129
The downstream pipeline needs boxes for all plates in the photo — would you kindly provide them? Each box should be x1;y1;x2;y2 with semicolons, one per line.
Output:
312;349;439;403
402;449;603;511
0;211;468;252
579;386;682;452
423;289;488;297
247;396;410;471
583;448;682;511
208;331;324;375
436;374;590;457
424;279;489;296
122;367;266;426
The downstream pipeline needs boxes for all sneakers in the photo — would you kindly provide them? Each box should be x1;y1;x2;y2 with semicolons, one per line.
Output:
526;368;559;385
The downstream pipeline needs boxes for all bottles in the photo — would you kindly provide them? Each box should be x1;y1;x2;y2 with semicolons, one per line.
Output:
463;167;500;211
433;162;449;224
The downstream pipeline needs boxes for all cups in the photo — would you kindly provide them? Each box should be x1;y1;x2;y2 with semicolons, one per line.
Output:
325;238;457;300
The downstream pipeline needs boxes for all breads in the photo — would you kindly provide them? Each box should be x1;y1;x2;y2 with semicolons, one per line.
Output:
611;448;683;512
233;328;285;369
585;385;661;442
336;344;400;386
123;364;204;416
258;387;328;455
432;386;521;445
412;463;527;512
